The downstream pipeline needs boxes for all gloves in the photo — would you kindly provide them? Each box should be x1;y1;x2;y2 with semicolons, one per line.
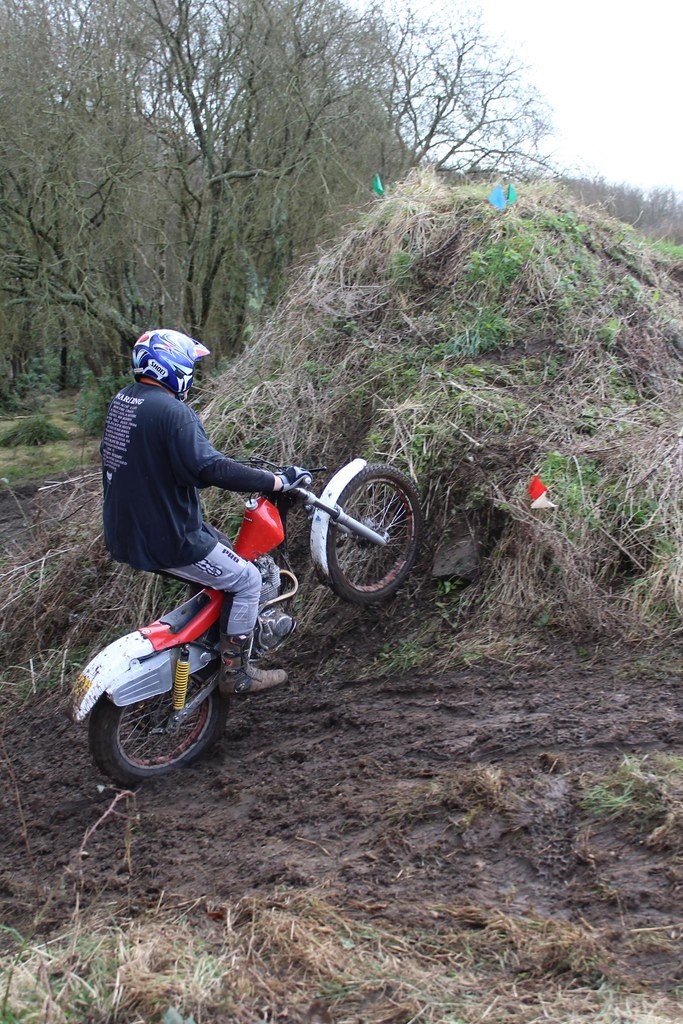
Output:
274;465;314;493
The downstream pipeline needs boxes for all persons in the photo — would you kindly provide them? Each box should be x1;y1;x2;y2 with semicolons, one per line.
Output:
99;328;313;696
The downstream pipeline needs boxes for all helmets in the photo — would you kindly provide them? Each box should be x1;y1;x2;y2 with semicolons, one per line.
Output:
132;329;203;398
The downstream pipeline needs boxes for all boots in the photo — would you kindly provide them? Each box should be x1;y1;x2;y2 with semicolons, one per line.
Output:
218;626;288;694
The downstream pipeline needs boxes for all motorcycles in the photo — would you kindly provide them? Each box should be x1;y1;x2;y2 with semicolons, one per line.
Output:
64;454;423;791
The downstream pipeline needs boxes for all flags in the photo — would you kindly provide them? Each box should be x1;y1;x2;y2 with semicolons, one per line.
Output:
372;173;384;196
507;183;518;207
527;475;559;509
487;183;506;210
